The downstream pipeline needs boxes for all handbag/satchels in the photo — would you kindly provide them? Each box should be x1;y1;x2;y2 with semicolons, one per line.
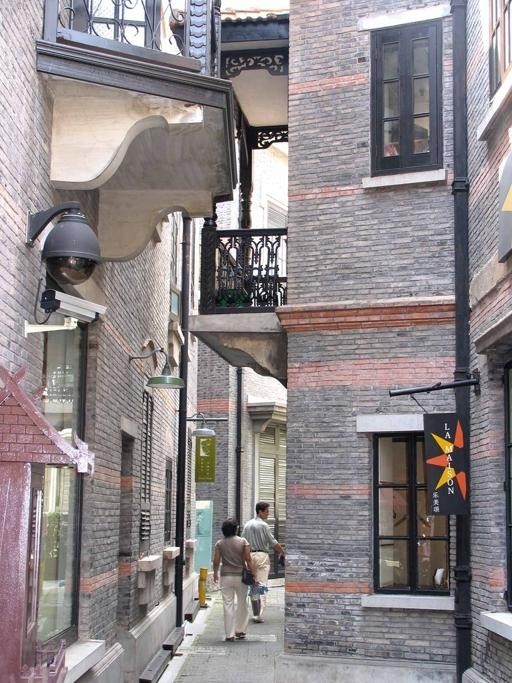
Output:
242;570;254;585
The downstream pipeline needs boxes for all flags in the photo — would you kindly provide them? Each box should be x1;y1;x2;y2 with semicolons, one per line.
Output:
194;434;215;484
422;409;471;520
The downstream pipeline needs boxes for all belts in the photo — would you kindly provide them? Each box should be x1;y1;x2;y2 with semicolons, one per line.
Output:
250;550;264;552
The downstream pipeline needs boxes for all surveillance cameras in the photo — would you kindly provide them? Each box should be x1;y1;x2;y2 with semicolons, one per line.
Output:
24;290;106;337
41;207;103;285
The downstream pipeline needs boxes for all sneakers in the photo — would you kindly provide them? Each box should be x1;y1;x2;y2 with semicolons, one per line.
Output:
254;616;265;623
226;633;246;641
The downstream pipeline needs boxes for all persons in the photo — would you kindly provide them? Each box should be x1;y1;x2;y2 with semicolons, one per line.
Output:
242;501;284;623
213;517;260;642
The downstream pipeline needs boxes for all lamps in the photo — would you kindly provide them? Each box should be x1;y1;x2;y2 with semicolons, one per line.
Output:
25;200;102;285
128;349;215;438
